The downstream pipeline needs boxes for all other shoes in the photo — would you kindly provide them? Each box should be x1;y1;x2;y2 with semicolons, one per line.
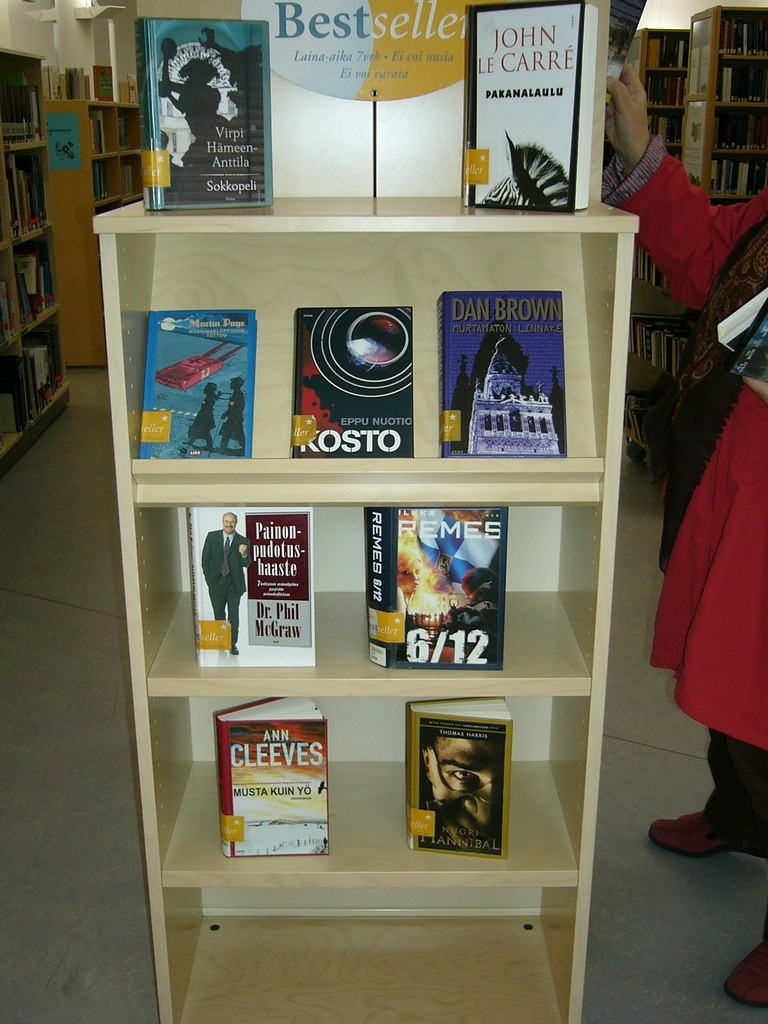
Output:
724;941;768;1007
648;810;768;858
230;644;239;655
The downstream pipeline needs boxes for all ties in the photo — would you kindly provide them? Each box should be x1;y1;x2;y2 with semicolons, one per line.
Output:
220;537;231;578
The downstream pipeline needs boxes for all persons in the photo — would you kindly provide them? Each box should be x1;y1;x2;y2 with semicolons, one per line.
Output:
605;63;768;1008
201;513;253;655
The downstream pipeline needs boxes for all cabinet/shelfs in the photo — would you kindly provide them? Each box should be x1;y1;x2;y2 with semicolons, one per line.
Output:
605;6;768;488
0;48;70;478
46;100;143;367
93;200;640;1024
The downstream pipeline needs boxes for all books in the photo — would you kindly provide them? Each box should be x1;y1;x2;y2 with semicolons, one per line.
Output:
186;502;315;668
434;288;569;462
363;504;509;670
137;307;256;460
290;305;417;458
135;20;276;212
404;696;513;860
3;62;140;432
458;1;602;212
212;696;330;860
602;10;768;448
